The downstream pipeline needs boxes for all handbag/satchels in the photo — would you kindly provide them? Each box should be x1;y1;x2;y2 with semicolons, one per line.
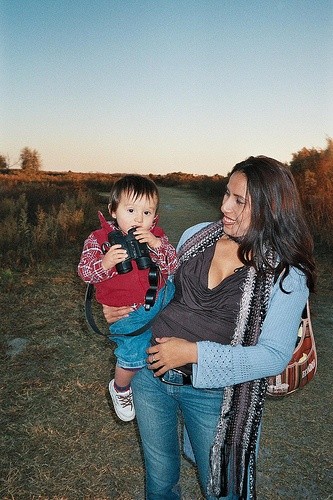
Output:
265;317;318;397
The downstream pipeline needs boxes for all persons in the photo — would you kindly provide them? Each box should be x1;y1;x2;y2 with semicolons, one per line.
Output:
76;172;180;422
100;152;321;500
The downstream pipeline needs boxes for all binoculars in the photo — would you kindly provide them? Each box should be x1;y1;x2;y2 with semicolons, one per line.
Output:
108;226;151;275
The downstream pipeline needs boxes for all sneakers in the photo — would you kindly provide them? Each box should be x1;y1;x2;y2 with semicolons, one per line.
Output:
108;378;136;422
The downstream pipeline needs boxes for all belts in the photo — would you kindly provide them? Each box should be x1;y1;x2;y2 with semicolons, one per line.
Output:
150;352;192;386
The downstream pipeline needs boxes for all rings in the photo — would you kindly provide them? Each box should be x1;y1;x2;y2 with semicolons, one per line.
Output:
152;354;156;362
